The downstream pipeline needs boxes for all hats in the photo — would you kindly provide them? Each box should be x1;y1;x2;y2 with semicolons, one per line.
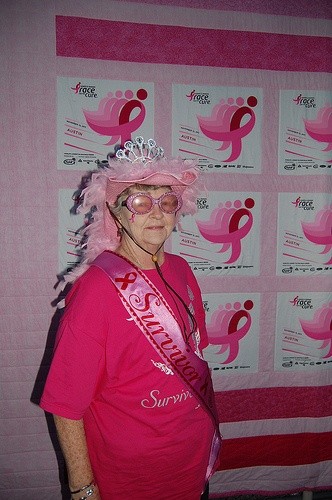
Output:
102;148;204;205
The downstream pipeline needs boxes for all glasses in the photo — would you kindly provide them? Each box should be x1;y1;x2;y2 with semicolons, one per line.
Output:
116;192;183;215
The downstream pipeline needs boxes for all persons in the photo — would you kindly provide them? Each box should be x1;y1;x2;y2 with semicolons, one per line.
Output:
38;136;222;500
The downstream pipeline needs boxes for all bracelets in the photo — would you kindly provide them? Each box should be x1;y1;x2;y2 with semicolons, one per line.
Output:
70;479;98;500
